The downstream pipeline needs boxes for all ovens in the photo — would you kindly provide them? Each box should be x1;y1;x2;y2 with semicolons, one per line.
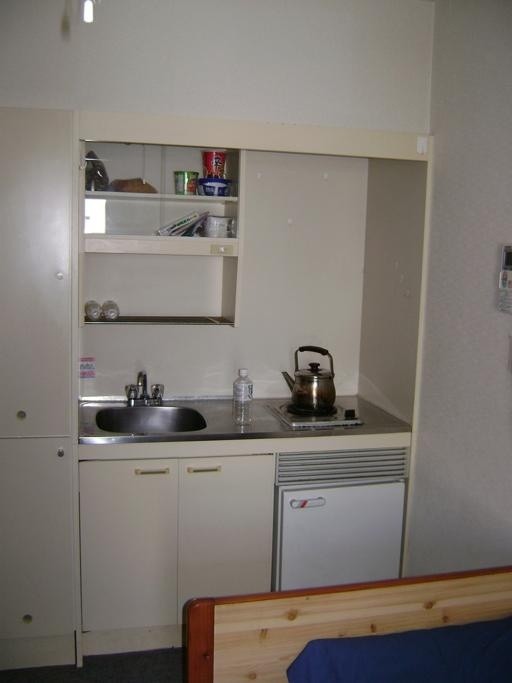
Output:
270;476;408;594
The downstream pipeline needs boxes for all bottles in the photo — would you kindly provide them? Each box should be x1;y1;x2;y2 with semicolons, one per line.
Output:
231;367;255;424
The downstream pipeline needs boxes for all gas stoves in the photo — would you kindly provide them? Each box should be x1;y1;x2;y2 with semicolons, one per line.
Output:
267;399;363;432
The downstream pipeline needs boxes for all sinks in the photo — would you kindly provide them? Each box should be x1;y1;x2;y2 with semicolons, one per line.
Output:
95;407;207;434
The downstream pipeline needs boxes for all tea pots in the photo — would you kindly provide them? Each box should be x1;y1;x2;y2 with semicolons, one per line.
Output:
281;344;336;412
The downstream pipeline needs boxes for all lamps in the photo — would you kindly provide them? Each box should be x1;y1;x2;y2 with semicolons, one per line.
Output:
83;0;94;24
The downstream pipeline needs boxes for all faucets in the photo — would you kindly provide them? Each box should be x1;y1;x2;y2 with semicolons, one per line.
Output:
136;371;148;402
